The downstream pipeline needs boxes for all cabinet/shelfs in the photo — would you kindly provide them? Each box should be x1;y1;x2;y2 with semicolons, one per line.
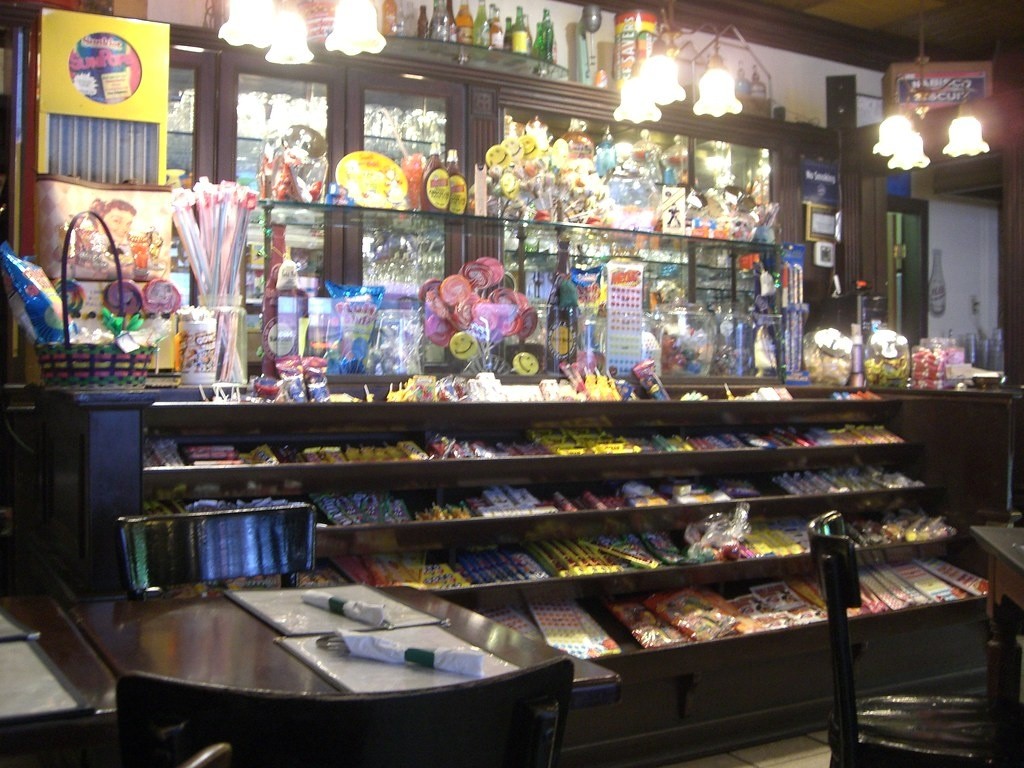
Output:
465;68;803;306
142;378;986;768
0;8;217;388
218;46;464;315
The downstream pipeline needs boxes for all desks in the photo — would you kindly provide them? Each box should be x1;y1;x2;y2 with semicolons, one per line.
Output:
969;524;1022;698
1;578;122;768
66;586;575;768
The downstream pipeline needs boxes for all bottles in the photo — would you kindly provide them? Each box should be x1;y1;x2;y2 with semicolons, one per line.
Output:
714;300;756;376
444;149;468;215
421;142;451;213
418;0;554;66
382;0;418;39
655;296;716;377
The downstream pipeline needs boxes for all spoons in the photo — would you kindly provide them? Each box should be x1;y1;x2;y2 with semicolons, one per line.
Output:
316;635;349;654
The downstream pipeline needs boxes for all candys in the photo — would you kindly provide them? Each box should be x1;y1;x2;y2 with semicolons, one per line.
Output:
368;306;426;375
486;135;607;224
417;256;540;376
50;278;179;337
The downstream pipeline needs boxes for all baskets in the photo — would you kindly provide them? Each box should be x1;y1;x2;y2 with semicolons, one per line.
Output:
33;211;162;393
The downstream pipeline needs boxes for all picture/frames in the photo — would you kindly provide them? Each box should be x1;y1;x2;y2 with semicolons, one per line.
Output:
805;202;839;244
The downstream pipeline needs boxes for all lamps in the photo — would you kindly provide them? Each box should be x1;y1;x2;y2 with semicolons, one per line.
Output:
612;0;743;124
870;4;989;171
215;0;387;67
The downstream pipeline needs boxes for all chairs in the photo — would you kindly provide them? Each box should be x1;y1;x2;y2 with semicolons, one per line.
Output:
810;509;1024;767
114;501;318;600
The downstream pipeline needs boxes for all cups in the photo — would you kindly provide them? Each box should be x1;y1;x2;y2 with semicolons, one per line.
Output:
179;319;218;387
954;328;1005;371
198;295;247;384
